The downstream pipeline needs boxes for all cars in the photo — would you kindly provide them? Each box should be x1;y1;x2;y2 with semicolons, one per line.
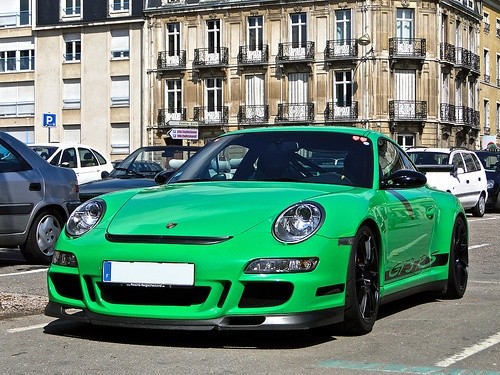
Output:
80;145;256;205
0;143;115;186
0;129;82;266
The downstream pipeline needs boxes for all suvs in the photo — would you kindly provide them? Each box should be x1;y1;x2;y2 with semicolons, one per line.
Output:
402;145;500;218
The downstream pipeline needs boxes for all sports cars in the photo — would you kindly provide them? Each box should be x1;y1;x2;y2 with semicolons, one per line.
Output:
45;123;470;335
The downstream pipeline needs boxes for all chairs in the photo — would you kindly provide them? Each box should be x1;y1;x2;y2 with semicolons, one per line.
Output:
253;156;294;182
81;153;93;166
341;156;373;187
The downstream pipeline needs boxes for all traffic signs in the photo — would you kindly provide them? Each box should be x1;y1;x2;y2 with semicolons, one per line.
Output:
166;119;199;128
166;127;199;140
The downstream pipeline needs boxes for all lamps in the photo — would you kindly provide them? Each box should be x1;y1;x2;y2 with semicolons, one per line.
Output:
358;34;371;46
